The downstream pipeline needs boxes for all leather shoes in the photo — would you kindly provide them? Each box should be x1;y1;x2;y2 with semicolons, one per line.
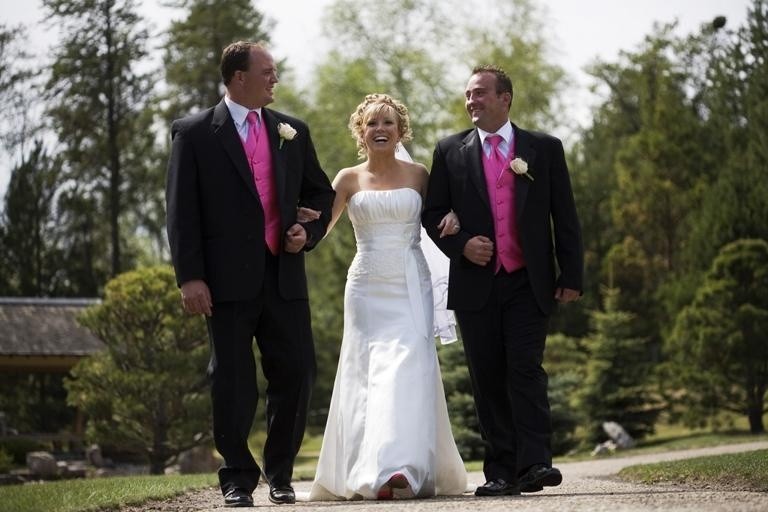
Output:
262;467;295;503
225;488;253;507
521;465;562;492
476;478;521;496
379;474;408;499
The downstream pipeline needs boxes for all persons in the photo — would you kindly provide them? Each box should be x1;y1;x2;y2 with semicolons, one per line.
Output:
163;39;339;509
294;90;466;503
419;65;588;498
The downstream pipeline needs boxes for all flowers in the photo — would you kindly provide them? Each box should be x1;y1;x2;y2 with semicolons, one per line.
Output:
511;158;534;180
277;122;298;150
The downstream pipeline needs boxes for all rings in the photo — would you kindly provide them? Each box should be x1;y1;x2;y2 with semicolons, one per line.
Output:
454;225;459;228
452;218;456;223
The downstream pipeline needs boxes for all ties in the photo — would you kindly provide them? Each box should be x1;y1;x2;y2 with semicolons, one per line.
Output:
245;111;259;156
484;136;506;181
